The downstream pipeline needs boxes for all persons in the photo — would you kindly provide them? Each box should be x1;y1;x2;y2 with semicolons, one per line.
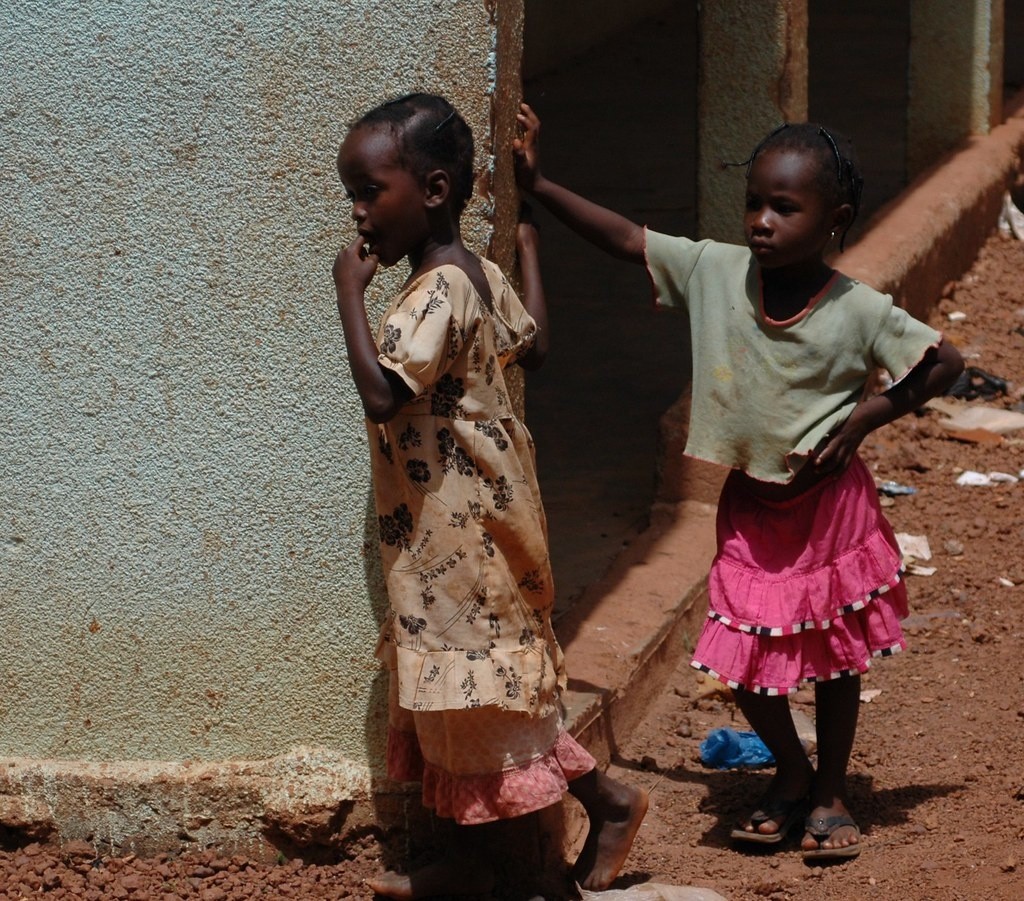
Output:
514;103;966;859
332;93;650;901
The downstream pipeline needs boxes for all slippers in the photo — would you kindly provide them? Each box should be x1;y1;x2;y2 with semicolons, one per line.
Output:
730;785;814;842
799;813;863;860
